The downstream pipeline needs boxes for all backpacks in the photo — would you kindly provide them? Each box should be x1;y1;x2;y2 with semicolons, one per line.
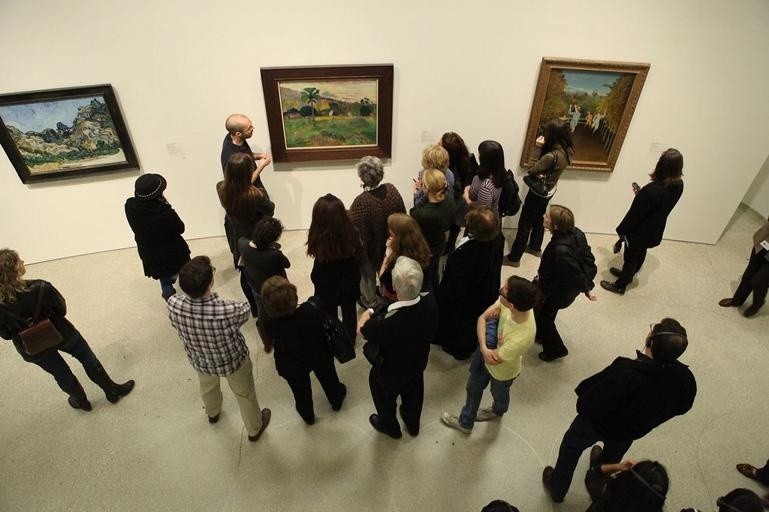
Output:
497;170;522;217
16;317;65;361
549;230;597;291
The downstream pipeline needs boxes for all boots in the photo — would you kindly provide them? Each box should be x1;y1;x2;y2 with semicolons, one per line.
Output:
255;319;274;353
56;375;92;413
85;359;135;404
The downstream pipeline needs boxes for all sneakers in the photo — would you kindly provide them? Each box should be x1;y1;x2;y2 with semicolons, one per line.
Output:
501;254;520;268
538;346;569;364
303;414;315;425
475;407;505;422
440;411;472;435
332;382;346;412
523;245;543;258
735;462;763;481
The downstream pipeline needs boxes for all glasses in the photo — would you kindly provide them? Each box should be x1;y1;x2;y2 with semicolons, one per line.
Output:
209;266;216;285
498;286;515;304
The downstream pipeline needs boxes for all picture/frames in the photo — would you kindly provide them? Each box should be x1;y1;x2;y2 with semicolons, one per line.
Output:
520;55;653;172
259;63;398;164
0;83;141;185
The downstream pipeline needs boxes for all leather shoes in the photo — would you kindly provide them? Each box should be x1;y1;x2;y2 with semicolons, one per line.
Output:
718;298;742;307
398;404;419;438
207;414;219;423
369;413;403;440
742;299;766;317
247;407;271;441
541;466;565;504
590;444;604;470
600;278;626;295
610;266;633;284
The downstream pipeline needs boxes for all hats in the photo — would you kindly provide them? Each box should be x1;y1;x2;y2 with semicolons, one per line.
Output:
133;172;168;202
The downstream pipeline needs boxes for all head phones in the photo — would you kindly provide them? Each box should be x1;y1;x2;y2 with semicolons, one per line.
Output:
646;332;687;348
716;496;742;512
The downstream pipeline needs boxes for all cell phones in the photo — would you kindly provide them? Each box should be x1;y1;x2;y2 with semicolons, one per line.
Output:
632;182;641;192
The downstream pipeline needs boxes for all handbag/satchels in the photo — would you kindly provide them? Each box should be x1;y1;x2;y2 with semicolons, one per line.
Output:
307;298;356;365
532;274;543;288
523;171;552;197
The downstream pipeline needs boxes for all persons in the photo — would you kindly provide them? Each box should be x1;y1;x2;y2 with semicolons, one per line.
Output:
0;249;134;412
